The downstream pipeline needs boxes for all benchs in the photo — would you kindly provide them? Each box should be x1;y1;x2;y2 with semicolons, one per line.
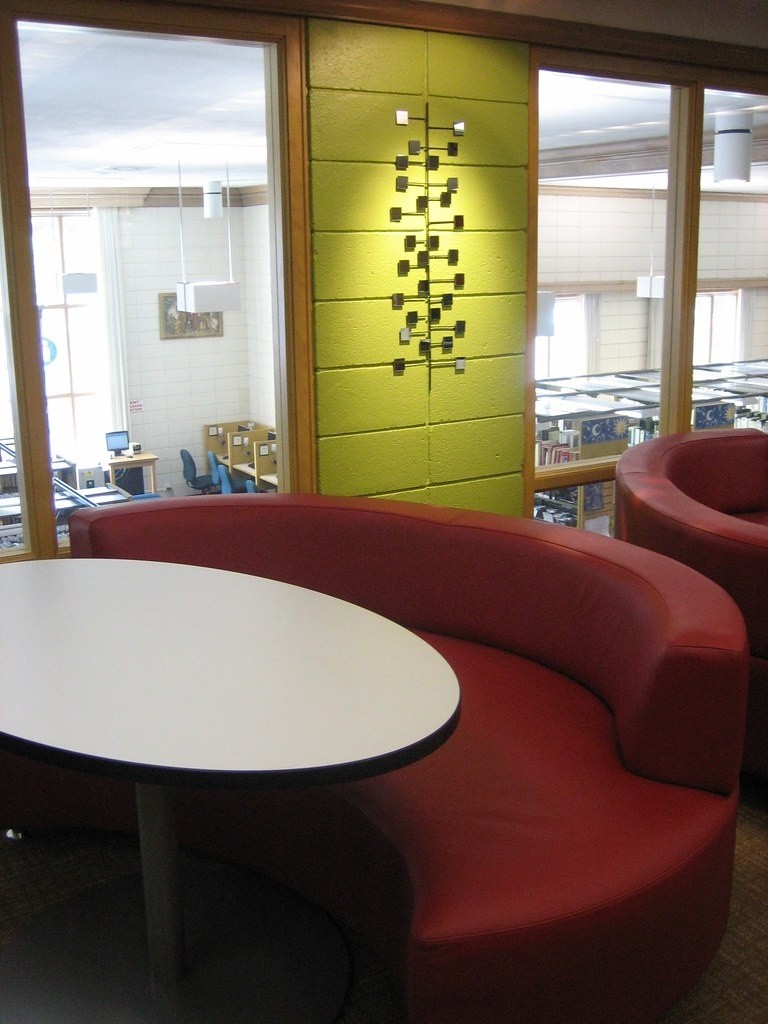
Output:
615;428;768;783
66;494;748;1024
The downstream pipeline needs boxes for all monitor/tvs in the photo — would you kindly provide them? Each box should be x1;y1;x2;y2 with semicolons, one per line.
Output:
238;425;250;432
106;430;129;456
267;432;275;440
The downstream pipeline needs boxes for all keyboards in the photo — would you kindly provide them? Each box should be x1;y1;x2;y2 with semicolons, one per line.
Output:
248;463;255;469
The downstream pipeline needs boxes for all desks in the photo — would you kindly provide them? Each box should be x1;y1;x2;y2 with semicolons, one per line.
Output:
104;450;159;494
0;557;463;992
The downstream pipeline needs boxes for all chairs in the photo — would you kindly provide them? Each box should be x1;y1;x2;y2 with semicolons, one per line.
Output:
208;451;244;493
202;420;279;494
180;450;214;496
244;480;268;494
217;465;248;495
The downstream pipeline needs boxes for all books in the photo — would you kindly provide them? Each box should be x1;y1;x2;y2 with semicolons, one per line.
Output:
535;438;580;466
734;412;768;433
628;425;645;446
537;426;580;447
0;515;21;526
0;474;17;494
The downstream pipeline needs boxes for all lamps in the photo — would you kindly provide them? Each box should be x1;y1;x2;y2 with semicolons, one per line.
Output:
203;181;223;219
713;132;754;182
636;186;664;299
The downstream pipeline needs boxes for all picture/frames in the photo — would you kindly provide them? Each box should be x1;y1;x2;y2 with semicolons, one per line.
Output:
159;292;223;339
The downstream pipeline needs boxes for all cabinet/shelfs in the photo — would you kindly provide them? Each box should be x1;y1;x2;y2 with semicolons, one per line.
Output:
534;359;768;538
0;454;132;549
